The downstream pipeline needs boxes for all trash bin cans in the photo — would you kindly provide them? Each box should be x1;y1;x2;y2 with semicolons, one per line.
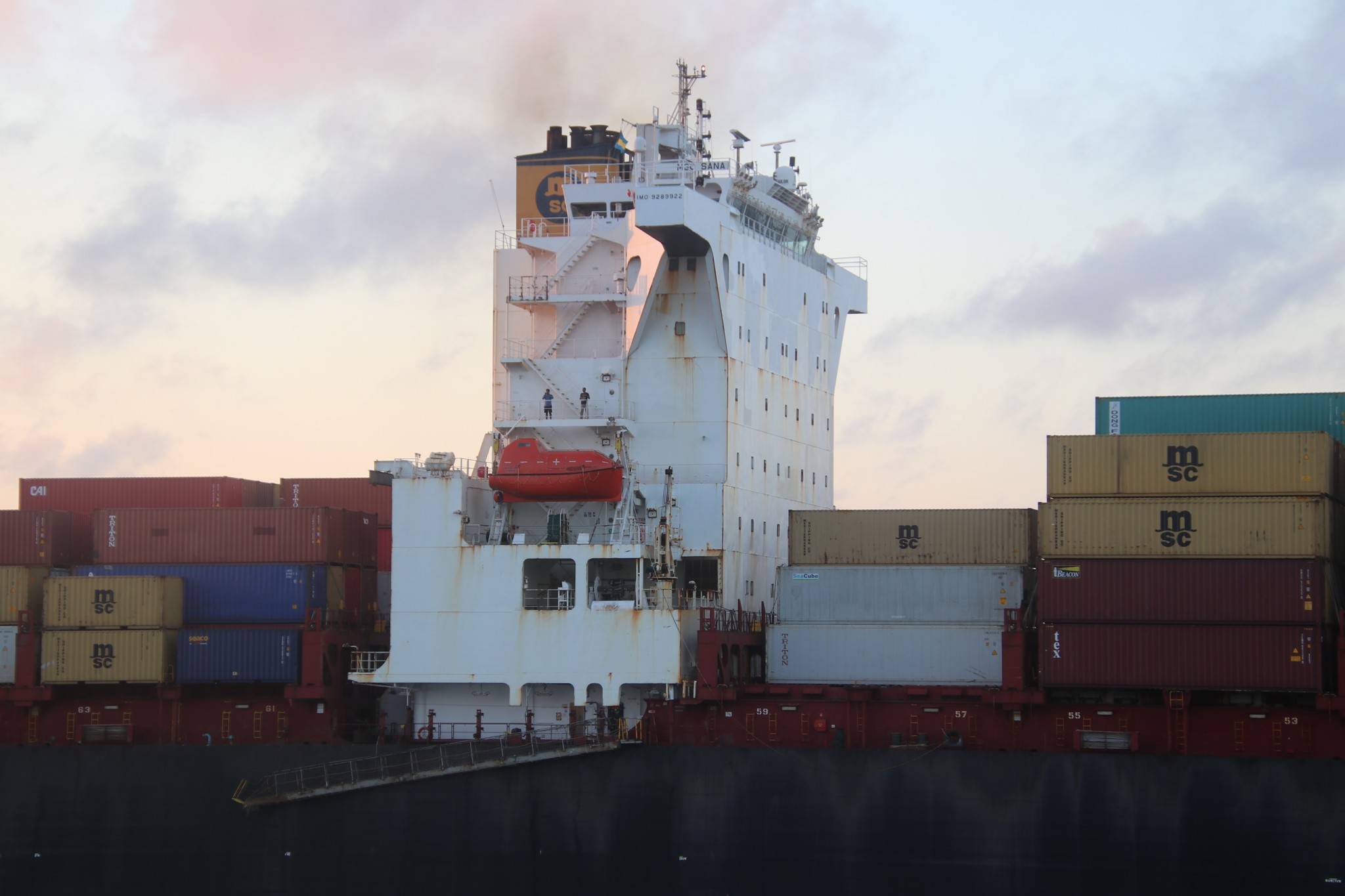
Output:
512;728;522;734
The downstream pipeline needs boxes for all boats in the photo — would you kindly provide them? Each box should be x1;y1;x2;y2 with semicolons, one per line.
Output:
488;437;624;503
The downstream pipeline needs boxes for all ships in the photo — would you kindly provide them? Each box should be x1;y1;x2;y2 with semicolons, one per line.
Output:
1;56;1345;896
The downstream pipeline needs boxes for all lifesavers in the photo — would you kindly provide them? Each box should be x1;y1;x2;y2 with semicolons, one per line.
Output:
640;528;645;543
418;727;430;739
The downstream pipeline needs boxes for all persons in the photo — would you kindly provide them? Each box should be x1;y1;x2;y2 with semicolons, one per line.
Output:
542;388;553;420
579;387;590;418
